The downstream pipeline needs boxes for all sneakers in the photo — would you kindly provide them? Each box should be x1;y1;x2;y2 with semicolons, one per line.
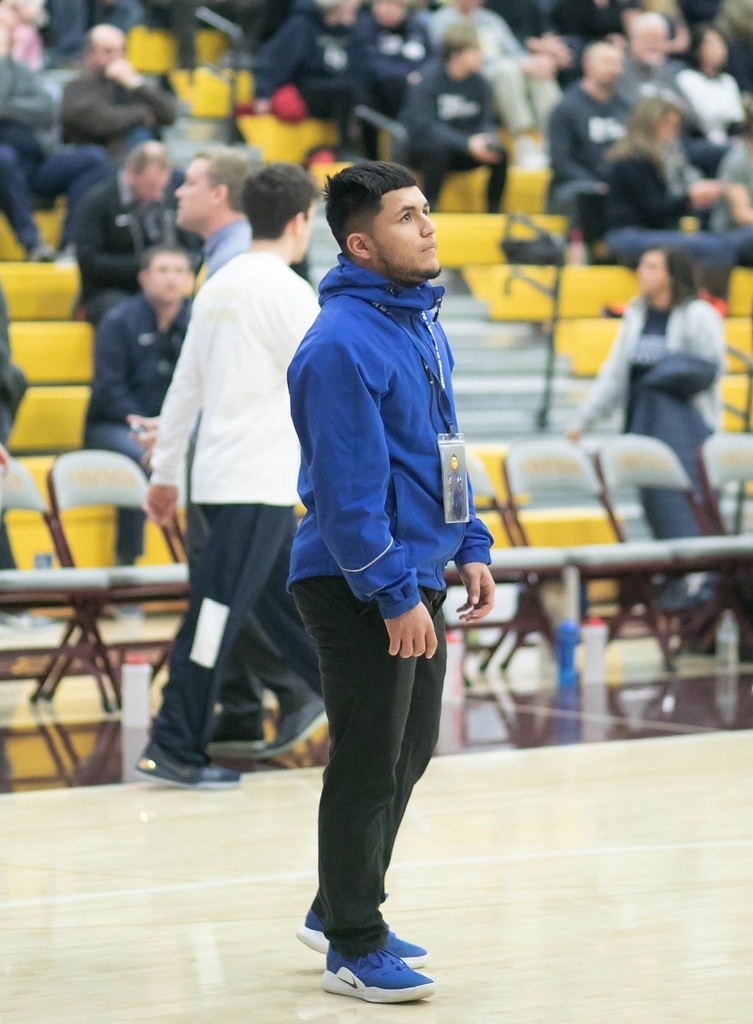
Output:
134;740;241;786
320;941;436;1003
296;907;428;968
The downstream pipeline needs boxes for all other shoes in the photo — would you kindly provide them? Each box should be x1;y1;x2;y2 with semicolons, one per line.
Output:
255;695;327;759
205;709;263;750
24;242;79;265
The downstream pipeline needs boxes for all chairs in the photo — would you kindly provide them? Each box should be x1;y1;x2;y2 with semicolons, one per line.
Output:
0;435;752;712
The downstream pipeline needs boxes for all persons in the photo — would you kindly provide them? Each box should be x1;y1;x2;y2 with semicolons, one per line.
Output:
0;290;30;569
129;165;324;788
0;0;753;323
80;244;196;568
449;455;464;520
287;161;495;1003
571;242;724;656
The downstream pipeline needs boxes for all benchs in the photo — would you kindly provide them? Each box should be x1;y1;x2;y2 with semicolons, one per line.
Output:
0;25;753;615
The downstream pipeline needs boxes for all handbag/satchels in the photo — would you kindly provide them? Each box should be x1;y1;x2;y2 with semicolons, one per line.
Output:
495;212;569;264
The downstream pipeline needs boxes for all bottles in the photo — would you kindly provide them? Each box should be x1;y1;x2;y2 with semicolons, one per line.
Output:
714;609;739;673
120;656;152;728
442;629;464;700
555;621;581;684
581;615;609;684
